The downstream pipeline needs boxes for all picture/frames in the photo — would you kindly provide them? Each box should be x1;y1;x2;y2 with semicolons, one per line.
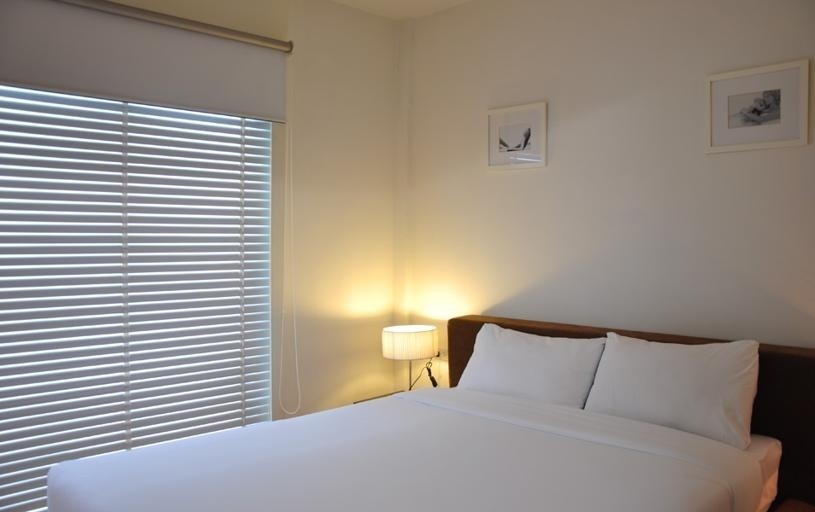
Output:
704;60;809;154
484;102;548;169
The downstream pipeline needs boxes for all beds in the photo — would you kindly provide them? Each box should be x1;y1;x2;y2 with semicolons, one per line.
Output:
43;312;815;511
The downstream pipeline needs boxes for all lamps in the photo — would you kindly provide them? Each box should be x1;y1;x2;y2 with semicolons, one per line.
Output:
381;324;439;392
